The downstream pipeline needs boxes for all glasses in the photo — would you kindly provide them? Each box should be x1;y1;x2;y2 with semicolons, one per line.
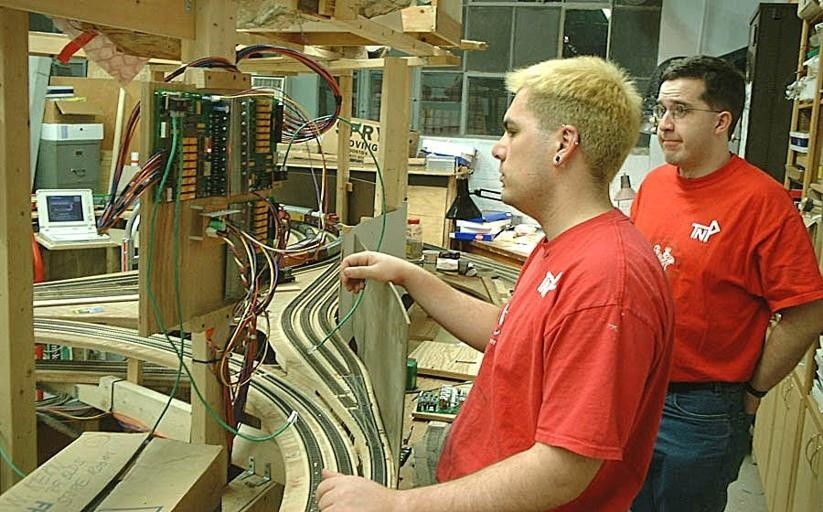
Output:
651;105;722;120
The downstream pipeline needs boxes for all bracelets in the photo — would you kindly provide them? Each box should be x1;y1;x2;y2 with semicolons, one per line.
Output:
742;380;769;402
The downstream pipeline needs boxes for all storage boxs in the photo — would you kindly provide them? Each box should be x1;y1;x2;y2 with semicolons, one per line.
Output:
42;100;103;124
1;431;225;512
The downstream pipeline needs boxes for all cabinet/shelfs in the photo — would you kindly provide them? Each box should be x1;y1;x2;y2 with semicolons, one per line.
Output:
34;140;101;194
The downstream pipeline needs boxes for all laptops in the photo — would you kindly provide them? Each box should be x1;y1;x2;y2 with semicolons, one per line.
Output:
36;188;110;244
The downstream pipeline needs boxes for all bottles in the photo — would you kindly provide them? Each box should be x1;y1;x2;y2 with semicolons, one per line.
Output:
406;217;425;264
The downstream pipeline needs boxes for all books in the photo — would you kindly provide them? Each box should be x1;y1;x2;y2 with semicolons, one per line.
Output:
447;210;512;241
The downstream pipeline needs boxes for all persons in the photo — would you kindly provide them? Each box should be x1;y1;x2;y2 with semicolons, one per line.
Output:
310;54;676;511
625;52;823;511
